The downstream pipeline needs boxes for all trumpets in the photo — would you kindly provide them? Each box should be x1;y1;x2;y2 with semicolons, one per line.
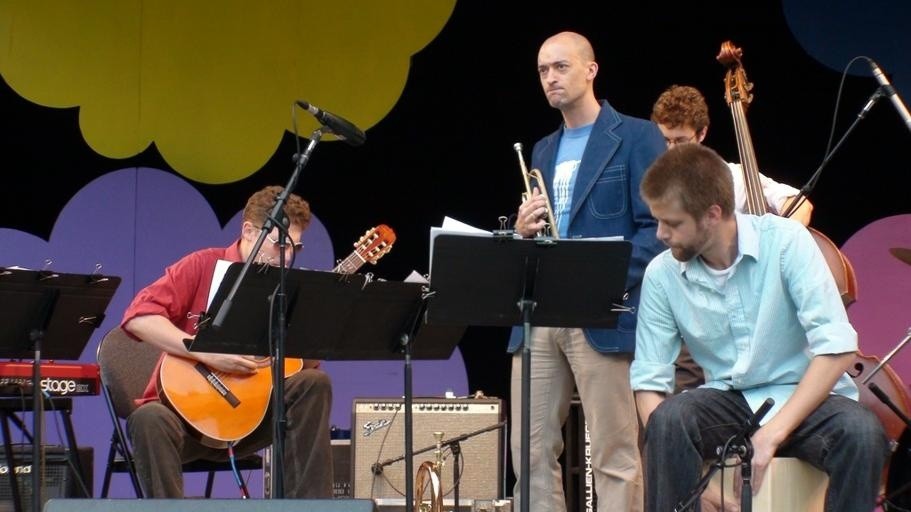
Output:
514;142;559;239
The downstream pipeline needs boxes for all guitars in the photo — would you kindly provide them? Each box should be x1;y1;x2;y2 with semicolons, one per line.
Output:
155;225;397;451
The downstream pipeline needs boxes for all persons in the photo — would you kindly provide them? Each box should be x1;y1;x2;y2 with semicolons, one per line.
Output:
118;186;336;499
627;141;892;512
507;31;669;512
649;86;818;227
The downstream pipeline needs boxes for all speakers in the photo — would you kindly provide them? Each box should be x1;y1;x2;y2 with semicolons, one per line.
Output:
350;396;507;505
0;445;94;512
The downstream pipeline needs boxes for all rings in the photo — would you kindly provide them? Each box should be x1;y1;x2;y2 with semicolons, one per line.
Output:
529;212;538;221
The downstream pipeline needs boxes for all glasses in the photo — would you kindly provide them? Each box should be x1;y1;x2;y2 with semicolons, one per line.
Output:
666;133;696;146
266;236;303;254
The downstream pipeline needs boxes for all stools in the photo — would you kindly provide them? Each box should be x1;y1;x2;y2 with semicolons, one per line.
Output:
0;396;94;512
699;448;830;512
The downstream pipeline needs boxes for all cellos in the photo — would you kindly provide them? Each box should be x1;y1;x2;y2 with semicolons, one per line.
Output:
718;41;906;507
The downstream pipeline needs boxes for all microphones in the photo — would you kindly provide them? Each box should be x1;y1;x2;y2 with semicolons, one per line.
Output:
371;463;384;475
866;58;911;130
297;101;367;144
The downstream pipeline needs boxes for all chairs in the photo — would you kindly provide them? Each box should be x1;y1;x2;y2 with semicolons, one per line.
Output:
94;322;273;500
